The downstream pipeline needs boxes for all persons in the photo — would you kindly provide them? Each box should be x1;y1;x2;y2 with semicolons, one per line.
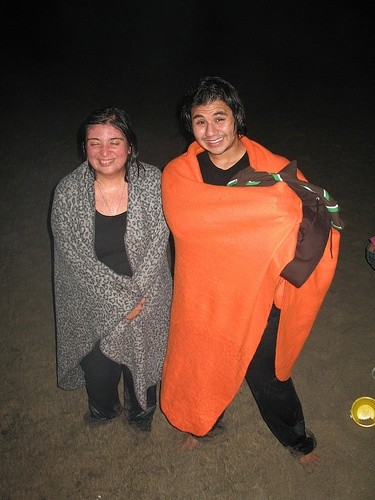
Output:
50;107;171;433
161;78;345;474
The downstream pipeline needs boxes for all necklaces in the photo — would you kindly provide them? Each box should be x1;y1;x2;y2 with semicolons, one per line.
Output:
94;175;125;216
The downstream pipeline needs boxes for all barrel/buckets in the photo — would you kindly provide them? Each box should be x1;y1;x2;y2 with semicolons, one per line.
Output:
349;396;375;429
